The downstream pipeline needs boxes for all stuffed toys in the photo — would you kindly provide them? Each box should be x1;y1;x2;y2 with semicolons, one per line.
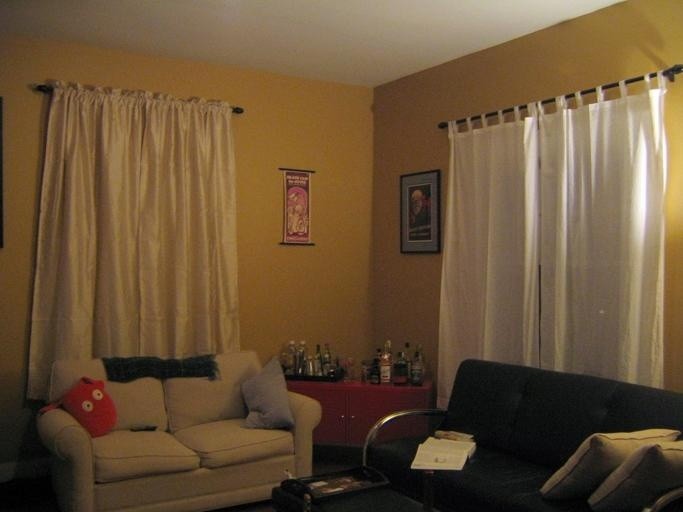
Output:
61;377;116;436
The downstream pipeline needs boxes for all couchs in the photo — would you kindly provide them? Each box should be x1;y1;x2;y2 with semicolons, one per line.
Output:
364;359;683;512
35;352;322;512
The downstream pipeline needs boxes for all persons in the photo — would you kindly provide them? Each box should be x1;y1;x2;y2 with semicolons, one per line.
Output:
410;188;432;227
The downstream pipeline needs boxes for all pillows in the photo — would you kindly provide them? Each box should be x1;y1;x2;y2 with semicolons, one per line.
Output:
540;429;683;512
49;352;296;437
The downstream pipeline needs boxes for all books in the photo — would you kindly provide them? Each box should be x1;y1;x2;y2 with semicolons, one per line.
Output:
410;435;477;473
434;429;477;445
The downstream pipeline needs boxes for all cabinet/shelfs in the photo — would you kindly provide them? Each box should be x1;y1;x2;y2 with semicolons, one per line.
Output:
287;374;433;448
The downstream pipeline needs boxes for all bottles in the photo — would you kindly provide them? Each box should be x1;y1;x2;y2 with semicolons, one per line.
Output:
362;338;425;387
280;339;342;378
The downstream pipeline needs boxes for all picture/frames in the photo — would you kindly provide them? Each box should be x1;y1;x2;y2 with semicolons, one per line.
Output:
400;169;440;254
